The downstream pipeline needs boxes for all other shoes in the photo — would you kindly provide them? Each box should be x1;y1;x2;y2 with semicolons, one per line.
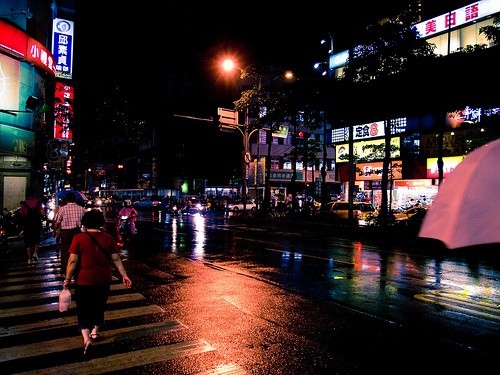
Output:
32;253;39;262
28;258;38;265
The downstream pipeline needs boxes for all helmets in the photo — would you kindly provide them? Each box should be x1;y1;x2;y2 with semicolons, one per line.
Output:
122;199;132;207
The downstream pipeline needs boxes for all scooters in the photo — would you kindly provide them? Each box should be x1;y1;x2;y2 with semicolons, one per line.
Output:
117;210;138;242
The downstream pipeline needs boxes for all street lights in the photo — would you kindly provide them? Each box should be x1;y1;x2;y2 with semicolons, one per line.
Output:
219;57;294;216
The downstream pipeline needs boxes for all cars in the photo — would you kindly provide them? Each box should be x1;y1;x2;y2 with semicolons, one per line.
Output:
134;197;163;208
392;205;430;221
224;198;260;212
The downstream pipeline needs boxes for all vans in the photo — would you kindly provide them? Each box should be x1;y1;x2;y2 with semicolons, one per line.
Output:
317;201;376;225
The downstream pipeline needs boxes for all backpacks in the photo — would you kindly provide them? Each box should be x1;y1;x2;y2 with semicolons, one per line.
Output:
22;200;42;227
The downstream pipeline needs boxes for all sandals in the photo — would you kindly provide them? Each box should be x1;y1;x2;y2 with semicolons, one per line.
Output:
83;339;93;355
90;334;101;341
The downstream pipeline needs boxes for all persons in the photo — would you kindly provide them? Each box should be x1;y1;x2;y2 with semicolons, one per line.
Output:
0;207;14;232
116;199;138;238
63;209;132;357
20;187;49;264
51;192;85;274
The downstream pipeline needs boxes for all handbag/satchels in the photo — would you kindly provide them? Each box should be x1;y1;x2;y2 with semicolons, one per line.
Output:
59;287;71;313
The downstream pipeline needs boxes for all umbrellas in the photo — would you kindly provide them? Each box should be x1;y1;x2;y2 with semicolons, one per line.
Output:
418;138;500;250
56;190;88;202
